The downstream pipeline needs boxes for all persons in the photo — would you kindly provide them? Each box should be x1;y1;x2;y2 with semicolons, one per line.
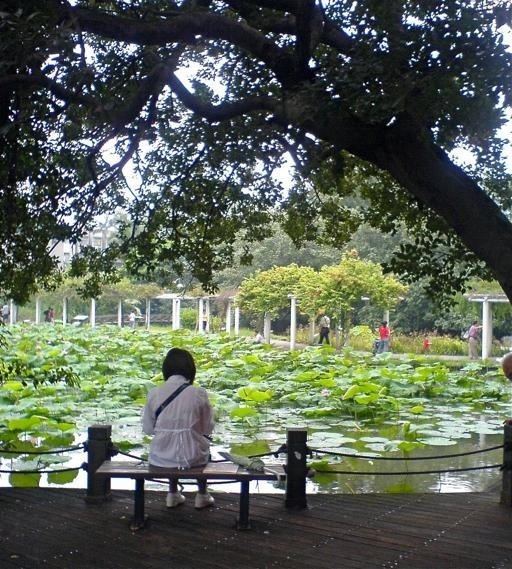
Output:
423;334;433;356
128;310;136;329
0;303;9;323
142;348;215;509
377;321;390;353
44;307;55;324
319;312;330;345
468;321;482;360
203;309;209;329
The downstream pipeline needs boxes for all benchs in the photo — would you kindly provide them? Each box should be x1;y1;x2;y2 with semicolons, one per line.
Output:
95;460;286;531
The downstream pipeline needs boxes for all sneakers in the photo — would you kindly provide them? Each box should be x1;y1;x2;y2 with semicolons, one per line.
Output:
166;492;186;508
195;494;214;509
